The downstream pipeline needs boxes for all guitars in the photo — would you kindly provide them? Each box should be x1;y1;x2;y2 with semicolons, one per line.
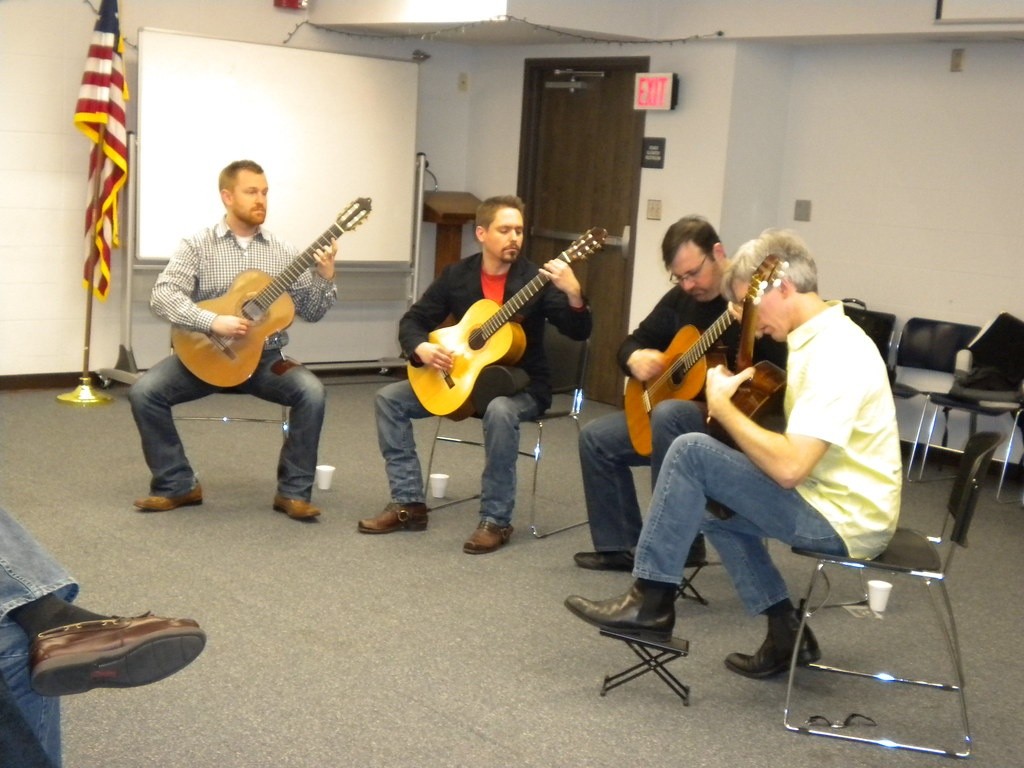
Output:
706;255;790;521
170;197;372;388
624;309;737;457
406;227;609;421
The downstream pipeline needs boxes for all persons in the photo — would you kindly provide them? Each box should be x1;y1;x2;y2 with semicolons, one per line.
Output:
573;216;784;572
358;197;593;554
564;228;902;677
0;505;206;767
127;160;338;518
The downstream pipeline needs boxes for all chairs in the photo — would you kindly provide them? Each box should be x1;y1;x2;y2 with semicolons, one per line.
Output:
168;297;1024;757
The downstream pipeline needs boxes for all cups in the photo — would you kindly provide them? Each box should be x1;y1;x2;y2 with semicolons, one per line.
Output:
429;474;450;498
867;580;893;611
316;465;335;490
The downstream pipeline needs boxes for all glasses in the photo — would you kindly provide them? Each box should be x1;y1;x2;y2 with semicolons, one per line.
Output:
669;255;706;286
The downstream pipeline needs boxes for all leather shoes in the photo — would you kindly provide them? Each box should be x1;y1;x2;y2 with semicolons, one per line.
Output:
29;609;208;697
273;493;321;519
133;480;203;511
564;585;675;642
723;609;822;678
463;520;513;554
358;503;428;534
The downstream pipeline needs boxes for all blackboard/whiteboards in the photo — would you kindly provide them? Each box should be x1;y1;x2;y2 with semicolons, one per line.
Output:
133;26;419;262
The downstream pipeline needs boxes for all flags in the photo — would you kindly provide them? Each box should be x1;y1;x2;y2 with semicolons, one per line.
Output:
73;0;128;303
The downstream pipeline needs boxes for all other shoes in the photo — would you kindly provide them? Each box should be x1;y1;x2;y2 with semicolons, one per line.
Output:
685;532;706;568
573;551;635;573
